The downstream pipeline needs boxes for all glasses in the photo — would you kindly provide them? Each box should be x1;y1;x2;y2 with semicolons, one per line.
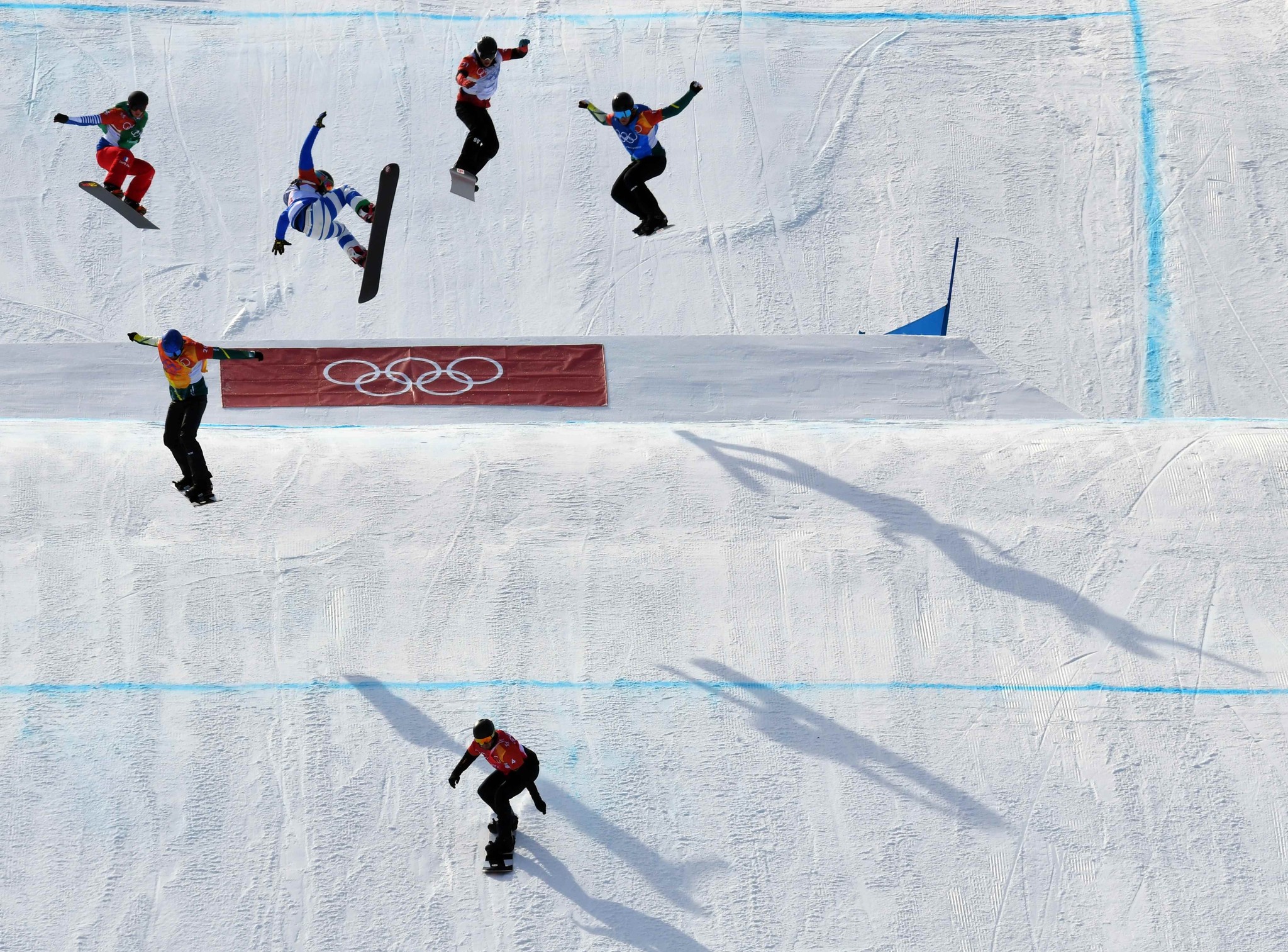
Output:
164;350;181;357
474;736;492;745
131;105;147;112
325;180;334;192
480;52;496;61
613;110;631;119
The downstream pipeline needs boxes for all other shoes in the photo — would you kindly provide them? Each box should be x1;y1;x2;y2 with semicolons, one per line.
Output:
123;197;146;214
359;205;376;223
186;480;212;497
175;477;194;490
475;185;479;193
633;212;667;234
104;184;124;199
475;176;478;183
347;245;368;268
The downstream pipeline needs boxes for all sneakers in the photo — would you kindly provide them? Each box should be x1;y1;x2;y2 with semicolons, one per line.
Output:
485;835;515;855
488;811;519;834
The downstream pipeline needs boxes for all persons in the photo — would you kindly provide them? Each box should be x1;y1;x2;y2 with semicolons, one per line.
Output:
455;36;530;193
577;80;704;234
128;329;263;497
271;111;375;268
448;719;546;852
54;90;156;214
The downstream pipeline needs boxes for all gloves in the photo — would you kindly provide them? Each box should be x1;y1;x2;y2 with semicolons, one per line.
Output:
271;238;292;255
463;79;476;88
534;798;546;815
54;113;69;123
448;773;461;789
519;39;530;49
255;350;264;362
315;111;327;128
579;100;590;108
689;81;703;95
128;332;137;342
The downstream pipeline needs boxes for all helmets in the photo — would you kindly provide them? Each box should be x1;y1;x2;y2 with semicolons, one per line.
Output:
473;719;495;739
479;36;497;53
161;329;183;353
611;92;632;112
315;169;334;188
129;91;149;107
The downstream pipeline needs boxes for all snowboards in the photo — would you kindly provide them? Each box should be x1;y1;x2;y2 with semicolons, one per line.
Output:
358;163;400;304
449;167;477;202
482;811;518;872
170;480;223;507
78;181;159;229
630;223;676;240
